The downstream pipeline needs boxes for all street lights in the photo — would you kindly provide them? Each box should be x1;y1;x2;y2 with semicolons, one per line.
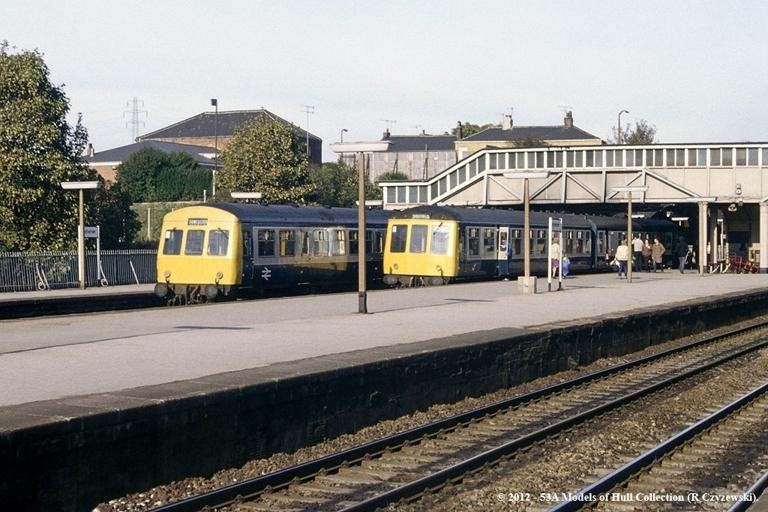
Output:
612;187;648;283
503;172;550;294
618;110;629;143
61;181;101;289
341;128;348;142
211;98;217;169
686;196;717;273
356;200;382;208
329;141;391;313
231;192;263;204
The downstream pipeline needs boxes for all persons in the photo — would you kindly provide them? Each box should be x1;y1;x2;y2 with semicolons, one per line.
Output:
616;239;629;278
649;237;666;273
632;234;643;274
684;250;695;272
562;253;571;278
550;238;560;276
642;238;652;272
676;236;689;274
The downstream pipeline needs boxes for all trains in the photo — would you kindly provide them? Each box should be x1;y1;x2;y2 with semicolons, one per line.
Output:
383;206;679;288
154;203;401;306
